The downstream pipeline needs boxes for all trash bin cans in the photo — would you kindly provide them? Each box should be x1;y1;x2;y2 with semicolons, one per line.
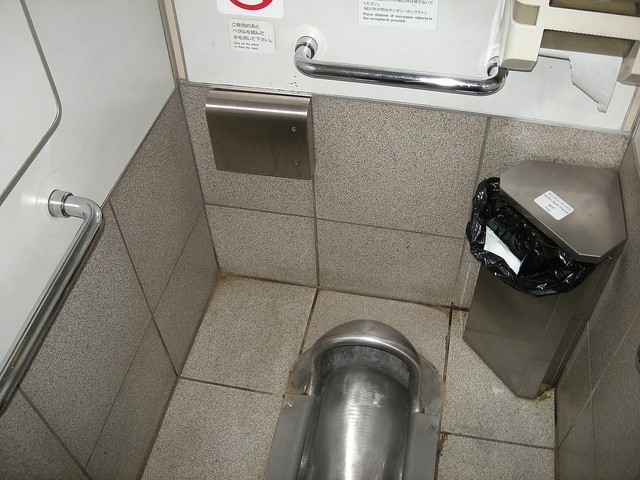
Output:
462;160;628;399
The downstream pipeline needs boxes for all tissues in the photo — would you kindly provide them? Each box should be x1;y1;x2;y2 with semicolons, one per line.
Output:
539;29;628;112
